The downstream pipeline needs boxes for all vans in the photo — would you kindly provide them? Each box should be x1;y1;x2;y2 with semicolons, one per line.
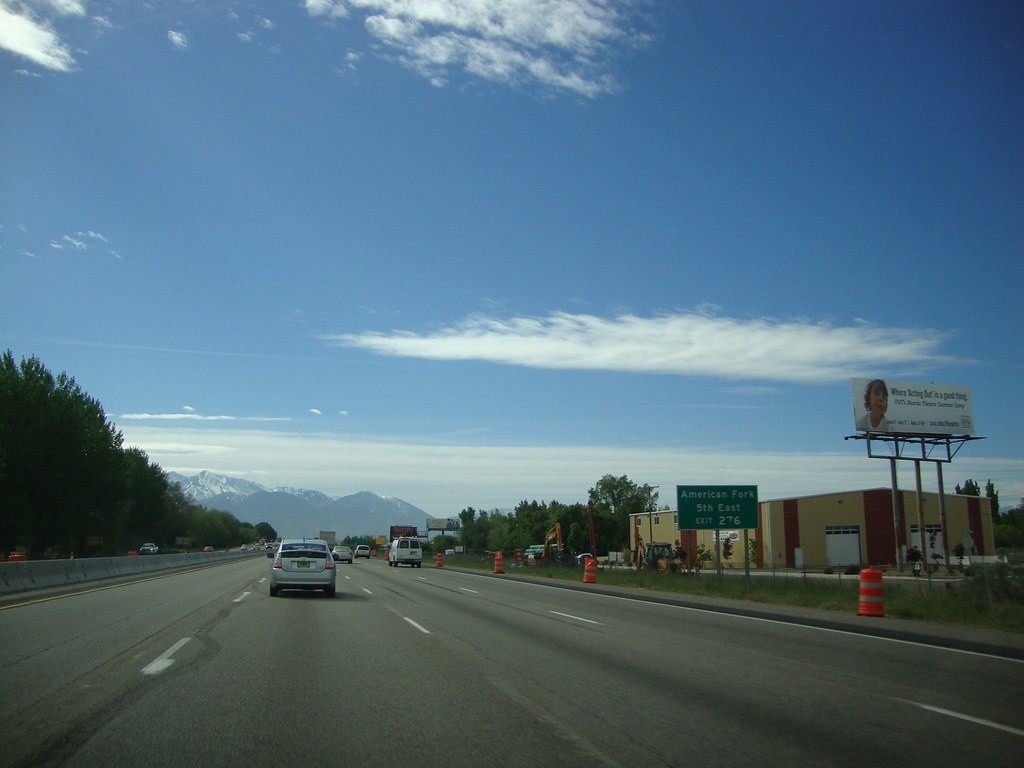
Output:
389;537;423;568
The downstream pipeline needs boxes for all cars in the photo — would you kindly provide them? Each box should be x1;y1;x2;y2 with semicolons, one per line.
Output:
51;552;78;559
203;547;214;553
140;543;159;555
240;537;280;555
266;537;336;599
332;546;353;564
354;545;370;559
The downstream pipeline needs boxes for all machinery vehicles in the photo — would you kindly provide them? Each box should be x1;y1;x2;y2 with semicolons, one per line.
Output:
526;522;564;567
634;535;682;574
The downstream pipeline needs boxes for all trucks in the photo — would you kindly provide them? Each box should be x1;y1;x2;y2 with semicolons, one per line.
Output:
319;531;335;551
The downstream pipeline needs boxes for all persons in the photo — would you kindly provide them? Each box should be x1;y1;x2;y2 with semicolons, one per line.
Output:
856;379;894;432
